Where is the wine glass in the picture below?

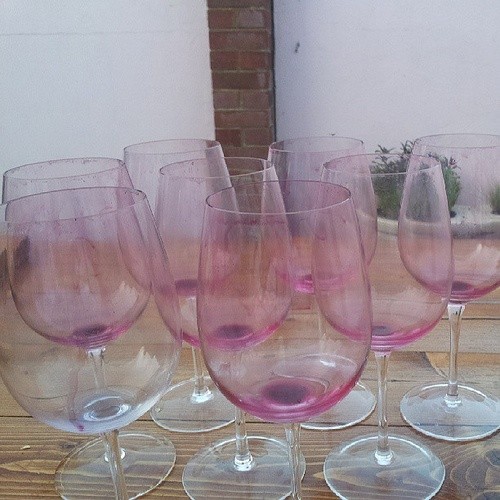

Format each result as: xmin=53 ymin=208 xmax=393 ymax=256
xmin=0 ymin=188 xmax=182 ymax=500
xmin=120 ymin=139 xmax=246 ymax=433
xmin=314 ymin=153 xmax=453 ymax=500
xmin=260 ymin=138 xmax=378 ymax=429
xmin=399 ymin=134 xmax=500 ymax=442
xmin=2 ymin=157 xmax=153 ymax=430
xmin=198 ymin=180 xmax=372 ymax=500
xmin=153 ymin=158 xmax=306 ymax=500
